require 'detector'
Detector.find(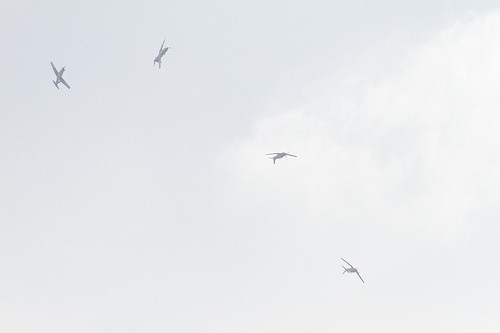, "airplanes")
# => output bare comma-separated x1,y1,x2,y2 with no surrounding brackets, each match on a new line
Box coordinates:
265,152,298,165
154,39,170,68
50,61,70,90
340,257,363,283
